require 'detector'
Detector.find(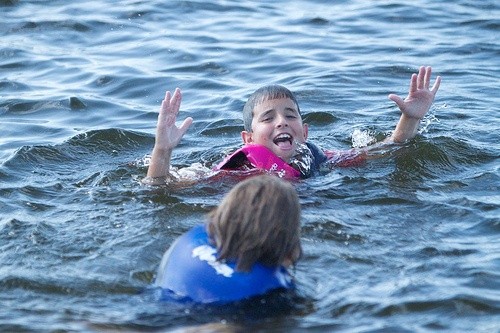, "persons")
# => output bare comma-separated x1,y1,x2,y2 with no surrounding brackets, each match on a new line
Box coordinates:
142,172,305,318
138,66,444,199
